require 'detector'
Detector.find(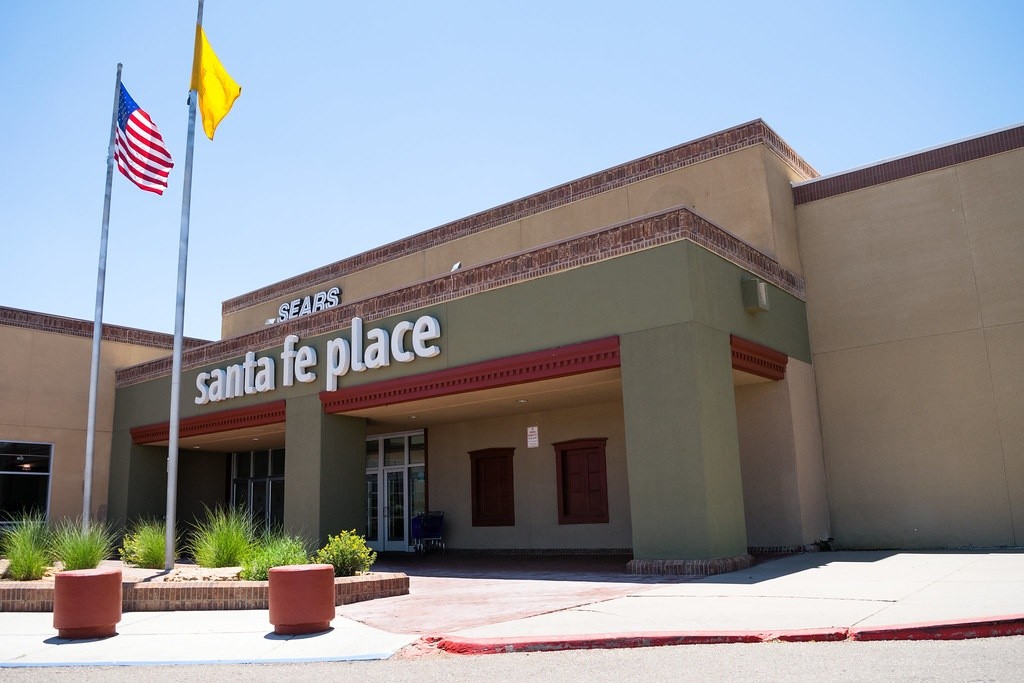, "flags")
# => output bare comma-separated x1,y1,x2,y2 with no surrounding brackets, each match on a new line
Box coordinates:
192,22,242,140
113,81,175,195
